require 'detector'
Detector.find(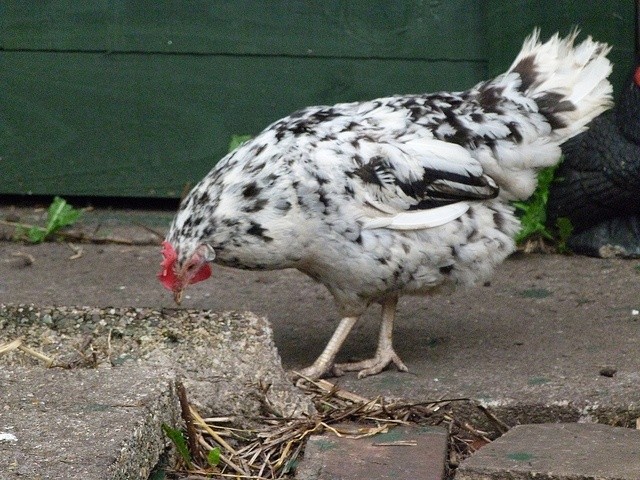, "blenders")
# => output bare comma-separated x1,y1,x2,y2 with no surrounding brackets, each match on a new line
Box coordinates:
155,25,616,387
514,63,640,254
